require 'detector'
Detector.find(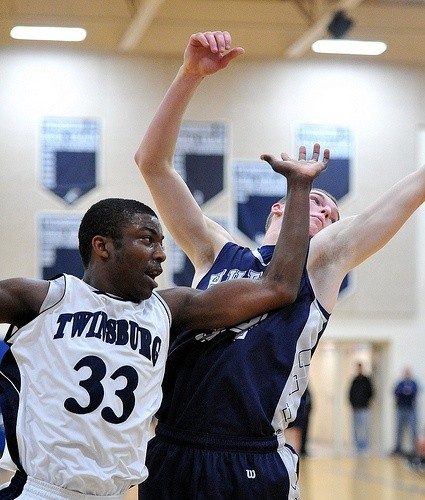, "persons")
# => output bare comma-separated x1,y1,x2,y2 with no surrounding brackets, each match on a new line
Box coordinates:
392,367,422,459
133,30,425,500
348,362,375,453
0,143,332,499
